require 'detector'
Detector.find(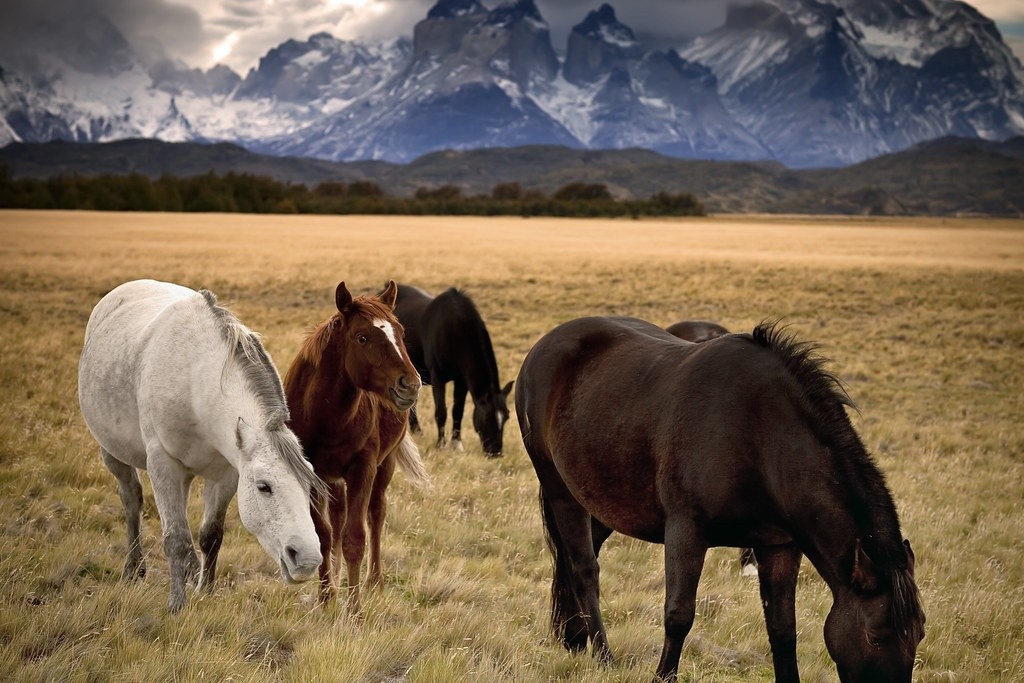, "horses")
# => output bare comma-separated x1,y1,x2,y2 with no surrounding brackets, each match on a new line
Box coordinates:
76,277,926,683
